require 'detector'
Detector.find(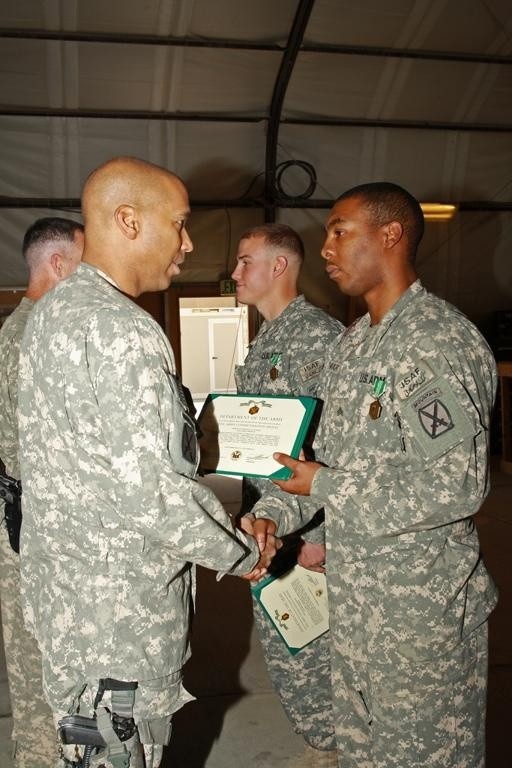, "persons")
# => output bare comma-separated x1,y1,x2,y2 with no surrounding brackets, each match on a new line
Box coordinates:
233,178,498,768
1,216,93,767
227,217,354,767
12,152,286,766
